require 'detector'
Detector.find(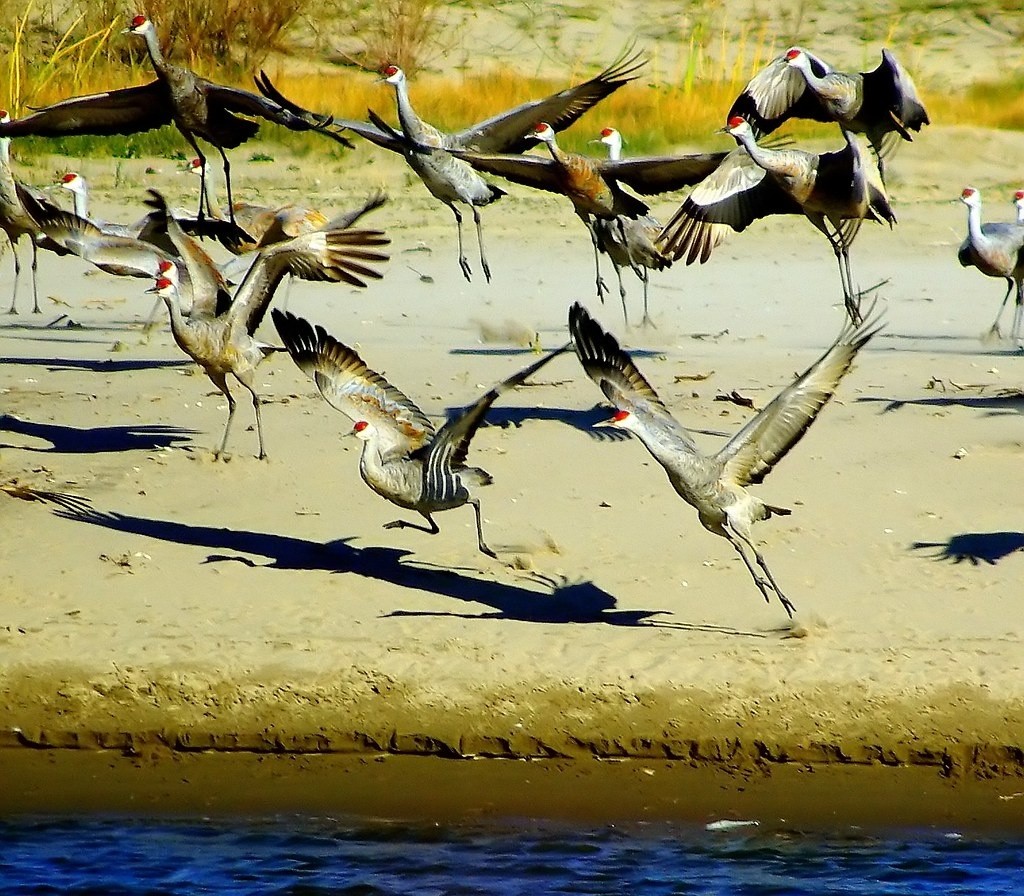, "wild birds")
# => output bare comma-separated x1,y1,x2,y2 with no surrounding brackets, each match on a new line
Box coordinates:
520,43,932,332
0,14,357,250
252,34,656,287
566,284,890,621
269,306,577,558
954,184,1024,344
1,108,394,466
